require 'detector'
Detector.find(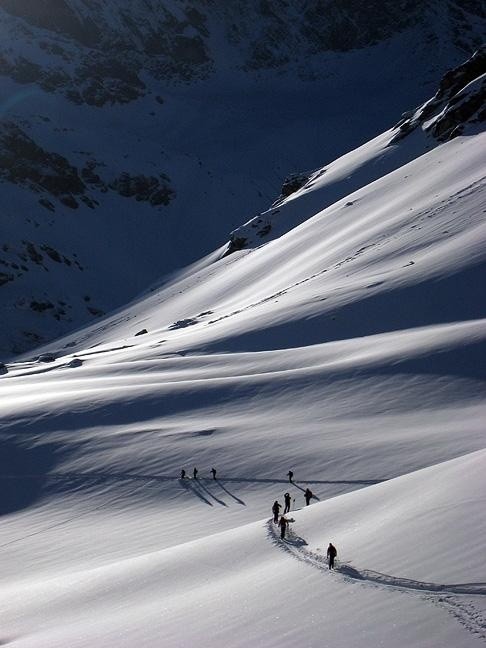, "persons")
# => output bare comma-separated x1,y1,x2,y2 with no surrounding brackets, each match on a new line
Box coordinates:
179,469,185,479
209,467,217,480
192,467,198,479
304,488,312,506
277,516,289,539
283,493,291,514
286,470,293,483
326,542,338,571
271,500,282,524
